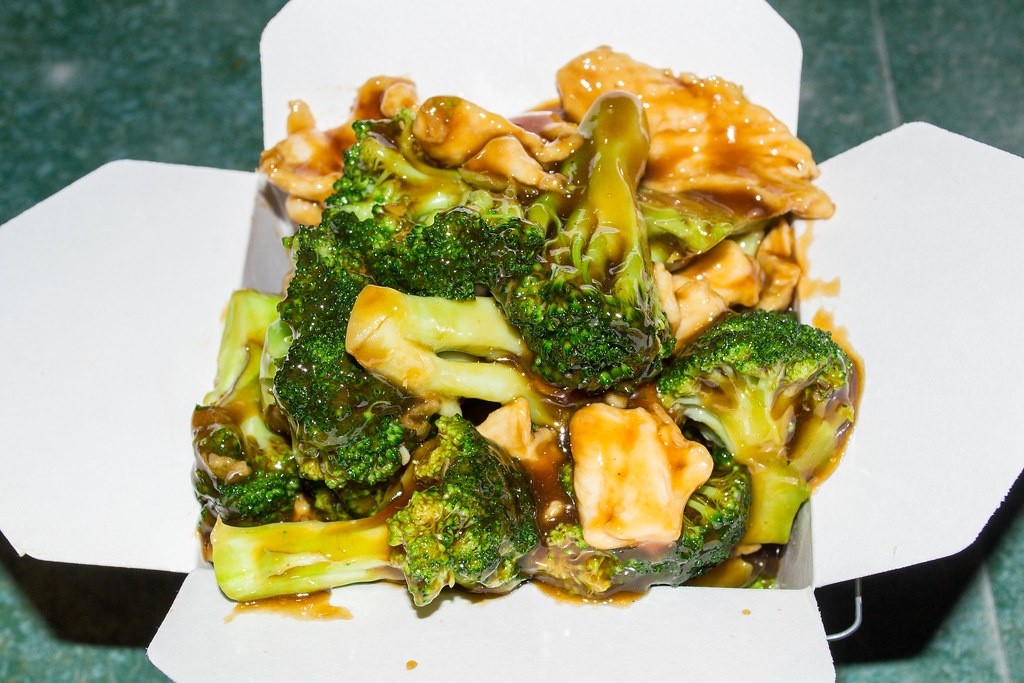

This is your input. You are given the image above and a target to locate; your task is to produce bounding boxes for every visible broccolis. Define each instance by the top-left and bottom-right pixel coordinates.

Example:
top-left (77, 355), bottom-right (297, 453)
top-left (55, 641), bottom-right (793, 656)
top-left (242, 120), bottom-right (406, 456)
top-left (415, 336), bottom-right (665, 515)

top-left (188, 108), bottom-right (855, 608)
